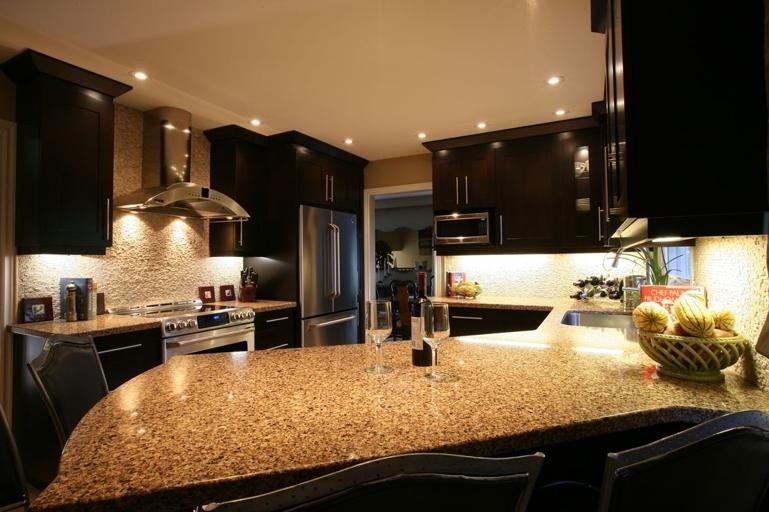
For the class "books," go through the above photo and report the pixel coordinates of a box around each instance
[59,276,97,320]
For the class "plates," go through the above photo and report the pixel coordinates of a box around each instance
[576,198,623,214]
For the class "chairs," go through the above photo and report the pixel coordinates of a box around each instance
[0,409,30,512]
[397,279,417,331]
[28,330,109,447]
[202,451,547,512]
[604,410,767,512]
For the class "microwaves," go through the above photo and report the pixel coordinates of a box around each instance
[435,212,493,247]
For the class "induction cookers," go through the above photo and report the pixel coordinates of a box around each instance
[115,299,249,318]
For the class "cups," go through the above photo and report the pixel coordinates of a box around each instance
[574,162,586,178]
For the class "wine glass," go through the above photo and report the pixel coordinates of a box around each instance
[366,301,392,376]
[421,303,450,381]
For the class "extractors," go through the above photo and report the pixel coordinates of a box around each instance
[114,106,250,222]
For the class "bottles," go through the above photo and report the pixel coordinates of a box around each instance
[65,281,78,322]
[411,271,433,366]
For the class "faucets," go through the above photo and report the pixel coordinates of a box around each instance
[610,238,694,268]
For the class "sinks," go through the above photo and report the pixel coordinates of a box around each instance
[560,307,639,331]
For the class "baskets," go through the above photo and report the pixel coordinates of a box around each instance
[638,330,745,384]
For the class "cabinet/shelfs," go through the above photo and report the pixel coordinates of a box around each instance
[204,129,364,210]
[424,301,489,344]
[12,327,162,434]
[255,299,297,353]
[591,0,686,235]
[423,129,505,213]
[2,48,133,258]
[506,118,580,250]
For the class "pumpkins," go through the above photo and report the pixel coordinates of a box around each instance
[711,305,736,332]
[631,300,670,334]
[706,328,736,340]
[663,321,683,338]
[671,294,715,338]
[684,289,706,305]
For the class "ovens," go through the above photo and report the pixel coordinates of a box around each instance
[162,307,255,364]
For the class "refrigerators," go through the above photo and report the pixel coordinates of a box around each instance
[299,205,364,347]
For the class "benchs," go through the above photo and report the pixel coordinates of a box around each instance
[377,299,394,340]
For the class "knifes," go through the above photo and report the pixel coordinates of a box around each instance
[240,266,258,288]
